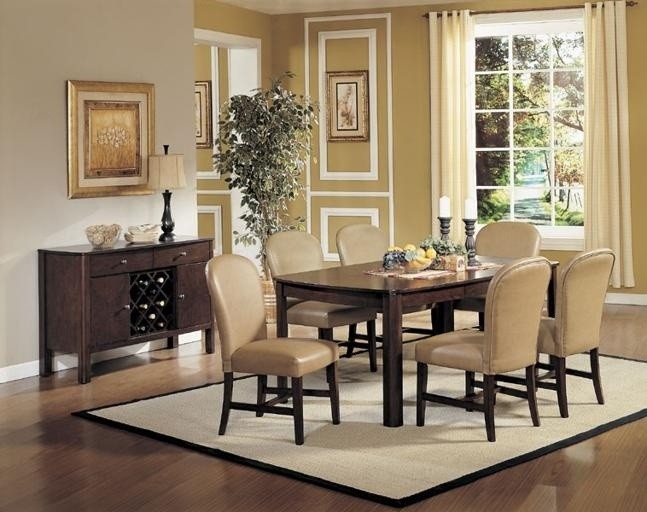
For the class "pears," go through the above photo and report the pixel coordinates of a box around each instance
[426,248,437,258]
[404,244,416,251]
[394,246,402,250]
[416,248,426,257]
[387,246,394,251]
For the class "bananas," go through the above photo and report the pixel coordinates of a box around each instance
[415,254,431,264]
[406,260,422,269]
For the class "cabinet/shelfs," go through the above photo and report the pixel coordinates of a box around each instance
[38,233,216,385]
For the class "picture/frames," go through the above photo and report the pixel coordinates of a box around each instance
[324,69,370,143]
[66,76,159,200]
[196,79,215,150]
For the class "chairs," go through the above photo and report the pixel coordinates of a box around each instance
[412,256,550,442]
[336,222,390,358]
[493,248,602,418]
[473,219,541,261]
[265,231,378,374]
[204,252,341,445]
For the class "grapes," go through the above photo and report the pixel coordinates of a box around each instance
[382,249,408,269]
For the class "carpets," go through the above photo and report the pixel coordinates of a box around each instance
[71,331,647,506]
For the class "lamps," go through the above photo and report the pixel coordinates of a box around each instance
[145,153,187,242]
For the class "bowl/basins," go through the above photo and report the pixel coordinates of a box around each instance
[395,259,434,274]
[86,225,123,250]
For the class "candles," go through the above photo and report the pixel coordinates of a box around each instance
[438,196,452,239]
[462,196,481,267]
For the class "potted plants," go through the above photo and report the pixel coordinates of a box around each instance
[209,69,320,325]
